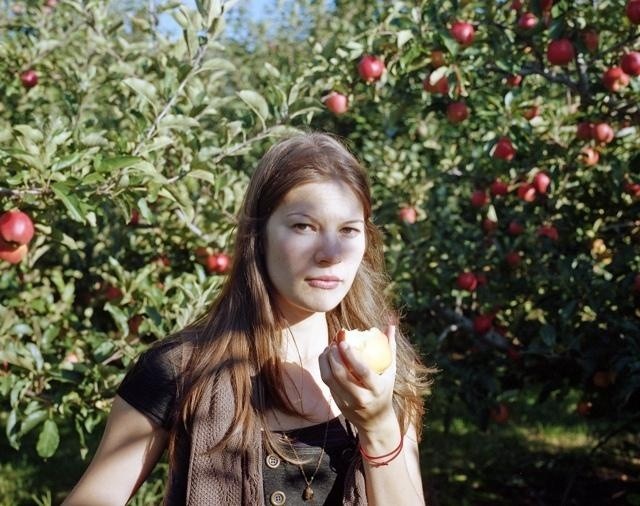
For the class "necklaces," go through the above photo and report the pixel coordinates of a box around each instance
[252,392,338,500]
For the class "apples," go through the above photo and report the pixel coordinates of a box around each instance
[1,0,640,425]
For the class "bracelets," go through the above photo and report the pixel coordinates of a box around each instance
[358,443,403,468]
[358,432,403,460]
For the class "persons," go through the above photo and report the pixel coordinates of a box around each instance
[59,130,445,506]
